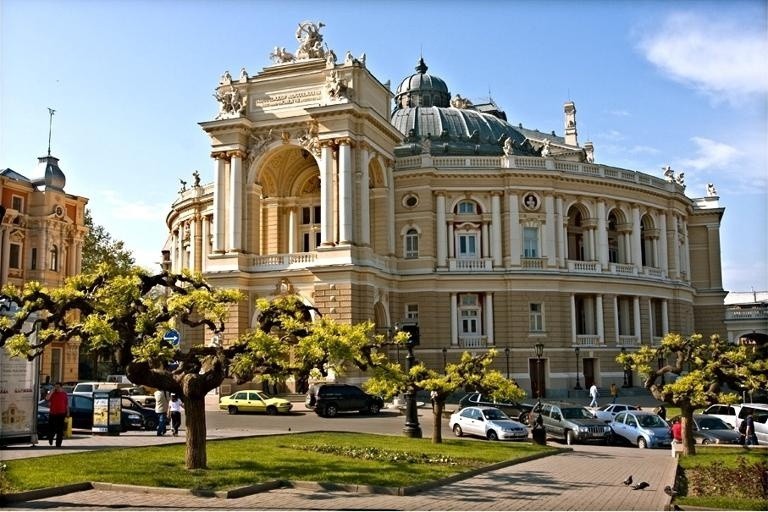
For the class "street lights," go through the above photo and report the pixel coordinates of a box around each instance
[573,347,584,390]
[533,337,545,402]
[504,346,511,380]
[622,346,632,388]
[441,346,449,377]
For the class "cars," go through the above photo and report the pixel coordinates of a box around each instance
[665,413,746,446]
[218,389,295,415]
[610,408,673,450]
[584,403,638,424]
[36,373,172,436]
[446,404,530,443]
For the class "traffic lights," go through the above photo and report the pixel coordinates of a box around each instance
[401,325,421,347]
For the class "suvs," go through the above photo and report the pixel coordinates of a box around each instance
[699,403,742,429]
[457,390,535,425]
[303,380,385,418]
[527,399,613,447]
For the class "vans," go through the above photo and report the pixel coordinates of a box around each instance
[735,402,768,446]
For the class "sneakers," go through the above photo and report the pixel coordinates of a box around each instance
[157,428,178,436]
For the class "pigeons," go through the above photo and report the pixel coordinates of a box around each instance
[664,486,678,496]
[620,475,633,486]
[630,482,650,490]
[674,505,684,511]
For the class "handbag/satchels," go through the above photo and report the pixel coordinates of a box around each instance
[63,417,73,439]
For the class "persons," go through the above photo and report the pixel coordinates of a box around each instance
[225,88,245,118]
[44,382,70,448]
[325,71,347,98]
[298,23,323,53]
[589,381,600,407]
[325,50,337,70]
[670,416,682,441]
[192,173,201,186]
[246,133,272,157]
[153,388,170,436]
[608,382,618,403]
[657,404,666,421]
[430,390,439,409]
[743,409,759,445]
[166,392,185,436]
